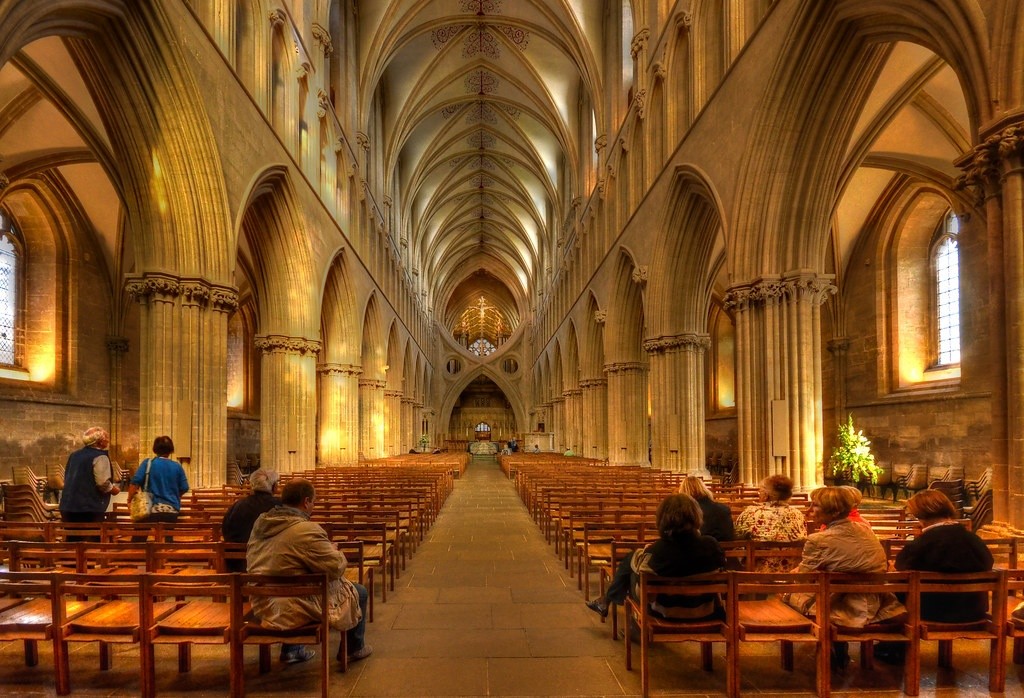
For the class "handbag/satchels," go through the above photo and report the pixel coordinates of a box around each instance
[130,457,152,522]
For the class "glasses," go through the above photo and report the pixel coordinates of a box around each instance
[758,490,765,495]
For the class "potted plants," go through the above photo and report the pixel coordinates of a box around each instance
[828,411,884,486]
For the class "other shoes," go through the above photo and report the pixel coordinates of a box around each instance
[874,641,905,666]
[830,642,850,668]
[337,644,373,662]
[620,627,641,644]
[280,649,316,663]
[584,600,609,617]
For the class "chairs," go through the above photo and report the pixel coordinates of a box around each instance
[496,451,1024,697]
[0,451,469,698]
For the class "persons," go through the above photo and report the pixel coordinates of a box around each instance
[246,478,373,663]
[433,448,440,453]
[534,445,540,453]
[127,436,189,543]
[734,474,807,583]
[585,493,727,636]
[785,486,888,669]
[820,485,871,531]
[507,440,518,452]
[409,447,419,454]
[59,427,121,542]
[875,490,994,666]
[222,465,281,575]
[564,447,574,456]
[679,476,739,566]
